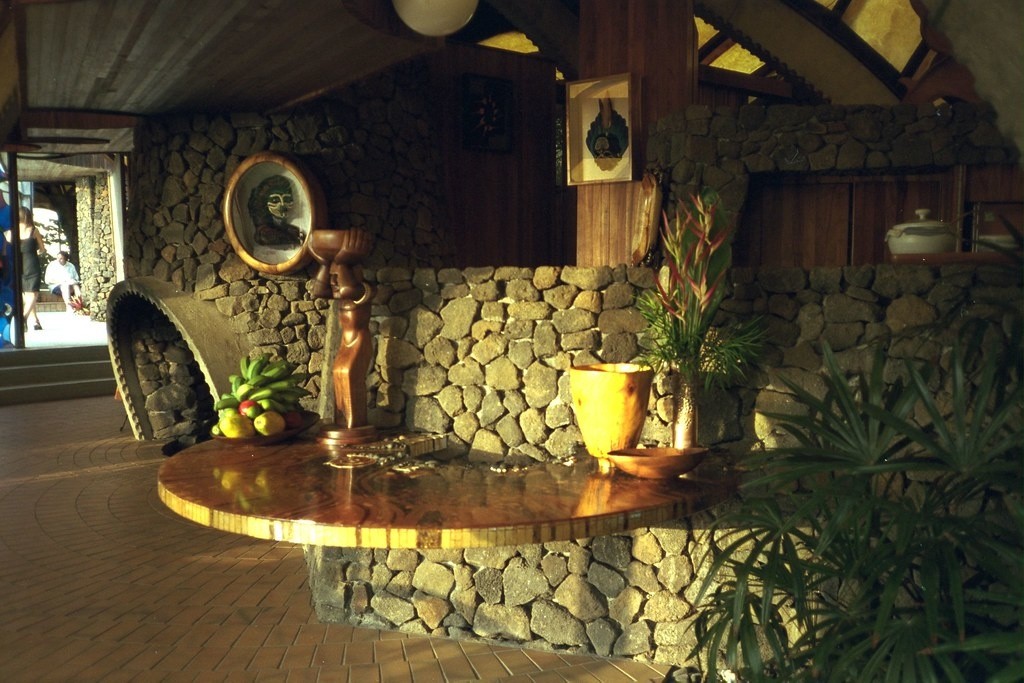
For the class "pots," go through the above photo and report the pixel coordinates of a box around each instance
[883,209,955,254]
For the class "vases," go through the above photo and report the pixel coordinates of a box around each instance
[672,380,699,448]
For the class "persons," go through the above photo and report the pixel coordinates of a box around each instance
[45,251,81,315]
[308,229,377,429]
[6,206,45,332]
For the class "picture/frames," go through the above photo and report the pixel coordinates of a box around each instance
[459,70,514,157]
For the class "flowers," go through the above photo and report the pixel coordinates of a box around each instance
[625,187,775,403]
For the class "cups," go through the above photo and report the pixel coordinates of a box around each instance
[569,363,652,472]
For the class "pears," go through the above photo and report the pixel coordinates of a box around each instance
[219,411,285,438]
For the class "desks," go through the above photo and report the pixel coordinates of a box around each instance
[156,427,739,551]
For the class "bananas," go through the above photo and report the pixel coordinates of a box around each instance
[212,352,313,415]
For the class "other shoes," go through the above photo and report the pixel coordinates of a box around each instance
[23,315,29,332]
[34,322,42,330]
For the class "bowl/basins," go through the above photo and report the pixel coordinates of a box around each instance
[608,447,709,478]
[313,229,372,264]
